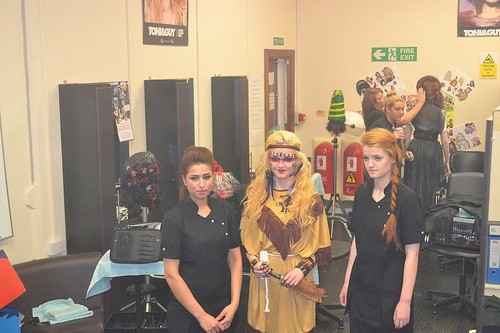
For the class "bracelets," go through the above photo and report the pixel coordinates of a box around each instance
[250,257,259,274]
[294,256,315,278]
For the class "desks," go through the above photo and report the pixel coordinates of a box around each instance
[316,240,351,330]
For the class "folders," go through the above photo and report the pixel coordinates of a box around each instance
[486,236,500,284]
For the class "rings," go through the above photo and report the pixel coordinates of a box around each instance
[262,269,265,273]
[265,274,267,277]
[264,272,266,275]
[261,265,263,270]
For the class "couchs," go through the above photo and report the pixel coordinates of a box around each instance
[7,252,102,333]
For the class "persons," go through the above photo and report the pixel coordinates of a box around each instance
[361,86,426,130]
[338,128,425,333]
[162,147,242,333]
[365,66,397,94]
[144,0,188,26]
[367,95,414,141]
[403,75,452,250]
[238,129,332,333]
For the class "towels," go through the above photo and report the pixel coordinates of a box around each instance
[312,172,325,196]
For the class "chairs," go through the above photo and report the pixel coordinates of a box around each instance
[421,150,484,321]
